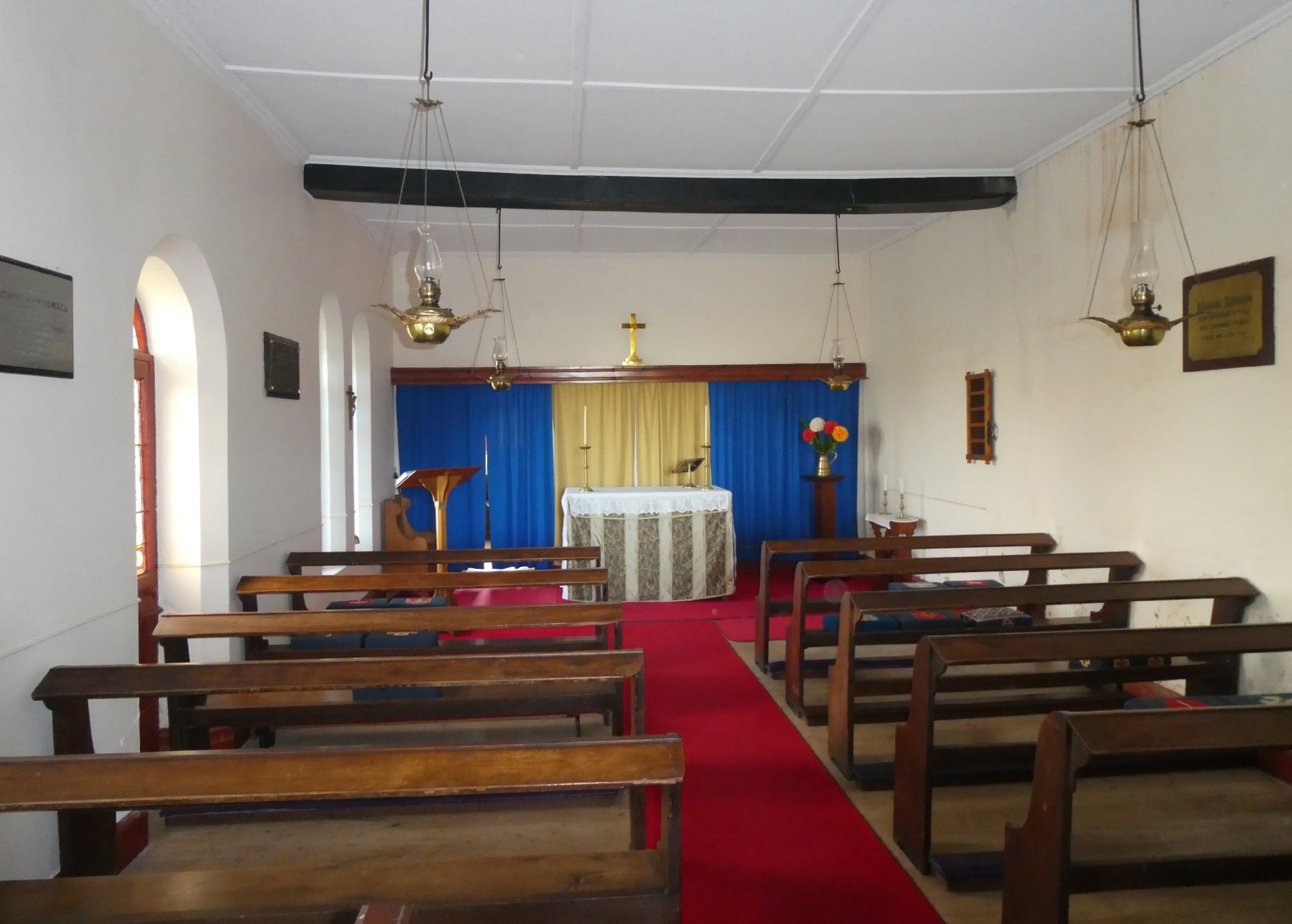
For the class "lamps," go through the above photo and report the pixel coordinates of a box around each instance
[370,1,503,345]
[472,206,534,390]
[812,212,869,390]
[1079,0,1209,347]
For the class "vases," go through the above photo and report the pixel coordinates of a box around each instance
[817,449,838,476]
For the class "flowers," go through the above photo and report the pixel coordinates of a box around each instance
[800,414,848,456]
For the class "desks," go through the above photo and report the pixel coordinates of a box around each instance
[561,485,737,603]
[866,513,919,582]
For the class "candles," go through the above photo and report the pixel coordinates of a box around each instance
[705,405,707,444]
[584,405,587,444]
[884,474,887,491]
[900,477,903,494]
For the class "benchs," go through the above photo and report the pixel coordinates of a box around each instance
[754,533,1291,924]
[1,545,685,923]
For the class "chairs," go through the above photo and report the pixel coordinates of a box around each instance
[384,493,437,598]
